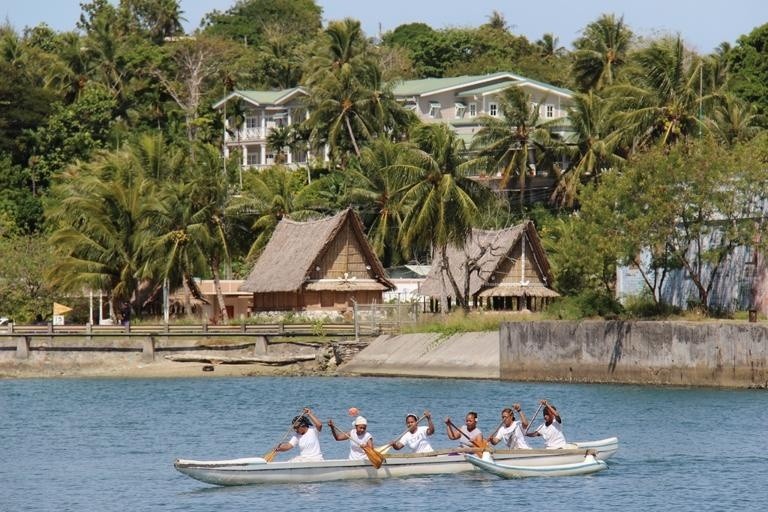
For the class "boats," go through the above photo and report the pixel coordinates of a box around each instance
[171,436,621,487]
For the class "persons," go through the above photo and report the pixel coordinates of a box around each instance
[522,398,568,448]
[120,301,130,326]
[327,414,375,459]
[442,411,483,447]
[274,406,325,462]
[487,402,531,450]
[388,408,436,453]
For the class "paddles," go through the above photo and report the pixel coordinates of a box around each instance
[447,421,494,458]
[264,409,307,463]
[332,423,385,468]
[374,416,425,455]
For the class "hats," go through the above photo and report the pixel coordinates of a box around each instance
[292,416,313,427]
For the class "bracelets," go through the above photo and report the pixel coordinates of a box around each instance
[517,408,521,412]
